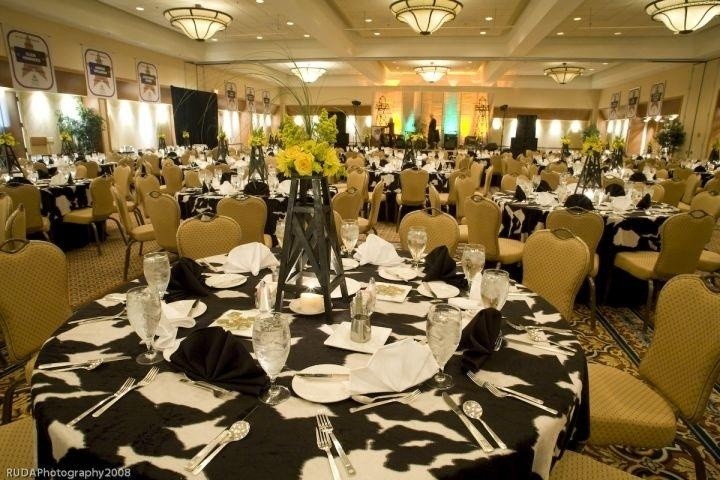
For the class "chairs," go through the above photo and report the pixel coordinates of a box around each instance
[0,412,38,479]
[455,150,720,328]
[273,148,456,261]
[548,449,655,480]
[0,236,79,416]
[587,273,719,478]
[1,126,273,267]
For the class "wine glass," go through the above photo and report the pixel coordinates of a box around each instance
[124,286,166,367]
[249,314,294,404]
[461,243,485,298]
[425,304,459,393]
[143,250,172,304]
[340,221,359,259]
[0,142,720,218]
[407,226,428,276]
[276,220,286,249]
[481,267,510,335]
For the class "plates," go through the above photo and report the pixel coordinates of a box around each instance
[163,257,486,364]
[291,362,353,403]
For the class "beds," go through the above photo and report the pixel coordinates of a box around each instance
[34,244,584,480]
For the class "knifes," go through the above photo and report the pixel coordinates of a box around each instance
[295,372,353,379]
[38,356,132,370]
[501,337,576,357]
[442,391,494,452]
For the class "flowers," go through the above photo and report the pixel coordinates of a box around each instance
[266,105,342,177]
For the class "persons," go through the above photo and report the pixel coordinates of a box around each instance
[386,119,397,147]
[379,127,388,147]
[427,114,436,149]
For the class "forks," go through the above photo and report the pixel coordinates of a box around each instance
[349,388,422,414]
[314,407,358,478]
[66,376,135,428]
[92,366,160,419]
[315,427,342,479]
[467,369,544,405]
[506,319,573,334]
[178,377,227,402]
[484,382,559,415]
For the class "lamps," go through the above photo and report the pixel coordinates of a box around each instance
[414,67,450,85]
[390,0,464,36]
[291,67,326,83]
[643,0,718,36]
[163,4,236,43]
[543,63,586,87]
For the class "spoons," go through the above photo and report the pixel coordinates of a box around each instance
[51,358,104,371]
[350,391,410,405]
[462,399,508,450]
[183,420,250,472]
[527,330,578,354]
